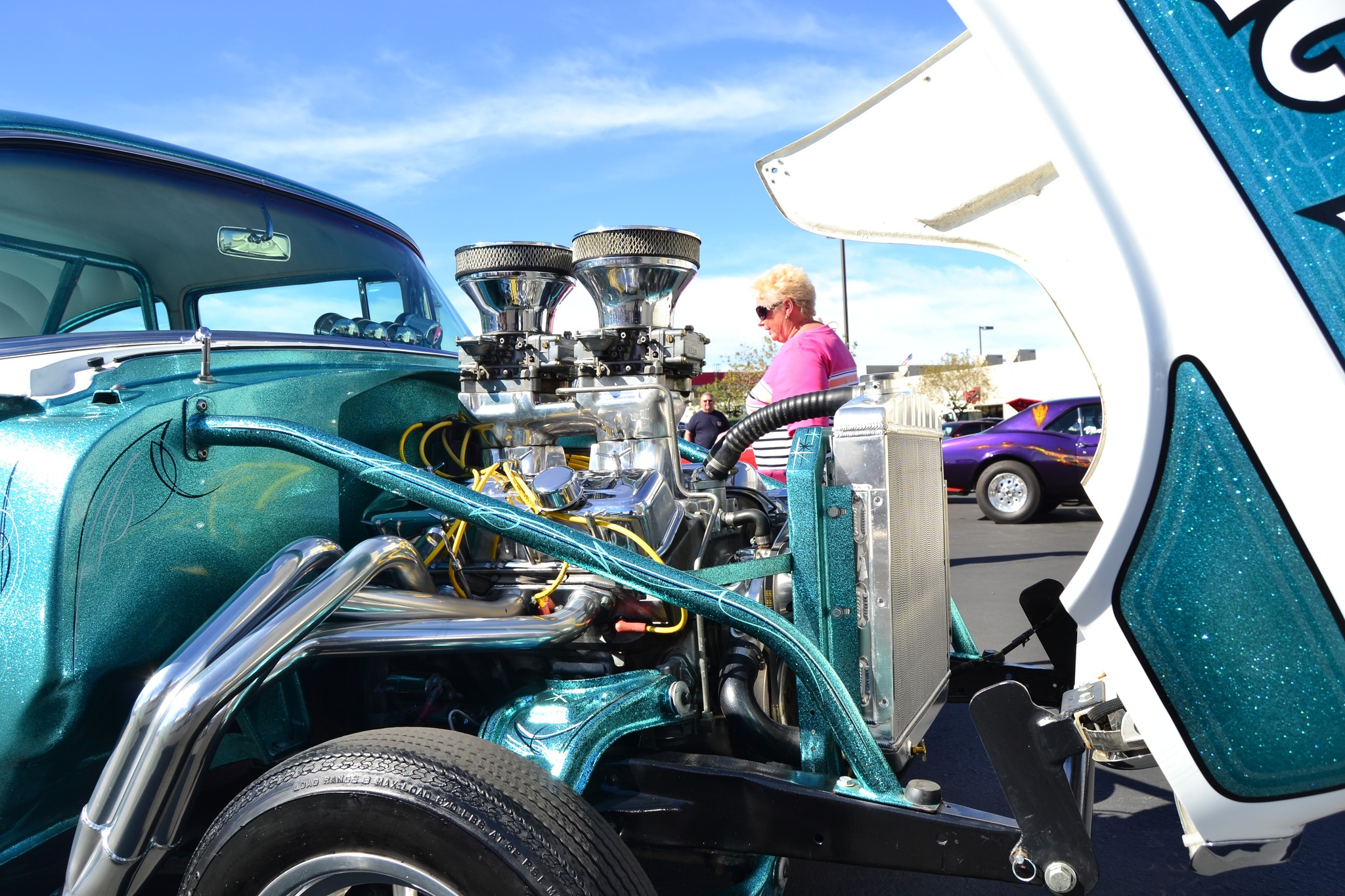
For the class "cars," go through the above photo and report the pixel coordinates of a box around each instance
[941,396,1103,525]
[0,0,1345,896]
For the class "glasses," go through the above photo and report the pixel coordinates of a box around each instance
[756,299,784,320]
[701,399,714,403]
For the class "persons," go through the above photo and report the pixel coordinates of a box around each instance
[744,263,858,470]
[684,391,730,450]
